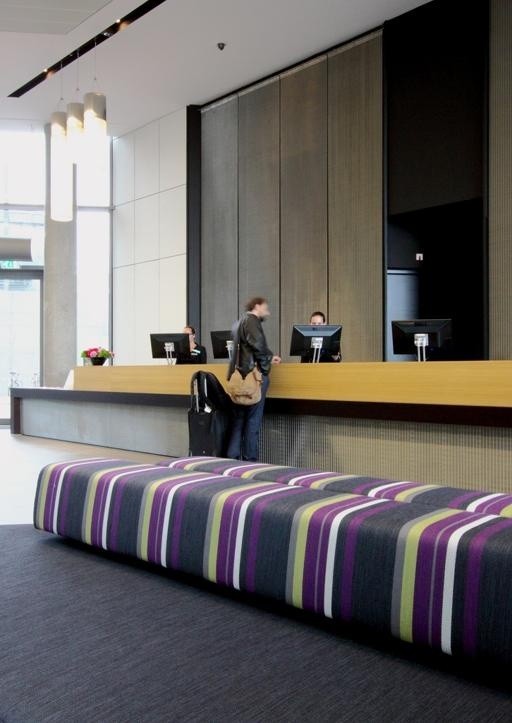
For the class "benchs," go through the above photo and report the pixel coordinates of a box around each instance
[32,454,512,675]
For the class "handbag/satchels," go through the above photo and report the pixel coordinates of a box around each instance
[225,361,264,406]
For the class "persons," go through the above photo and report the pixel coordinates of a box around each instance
[223,296,282,462]
[174,326,208,364]
[299,309,344,362]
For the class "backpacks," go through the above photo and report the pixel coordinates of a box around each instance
[190,371,233,414]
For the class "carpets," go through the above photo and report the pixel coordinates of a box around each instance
[0,523,512,722]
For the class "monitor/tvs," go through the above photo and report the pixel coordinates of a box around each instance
[211,330,233,359]
[150,334,191,365]
[290,325,342,363]
[391,318,452,361]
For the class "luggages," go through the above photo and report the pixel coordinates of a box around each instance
[185,373,229,459]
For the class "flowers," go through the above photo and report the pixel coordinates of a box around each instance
[81,347,114,359]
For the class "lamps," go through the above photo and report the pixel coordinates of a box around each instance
[50,37,106,141]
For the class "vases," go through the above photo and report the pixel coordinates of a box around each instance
[90,357,106,365]
